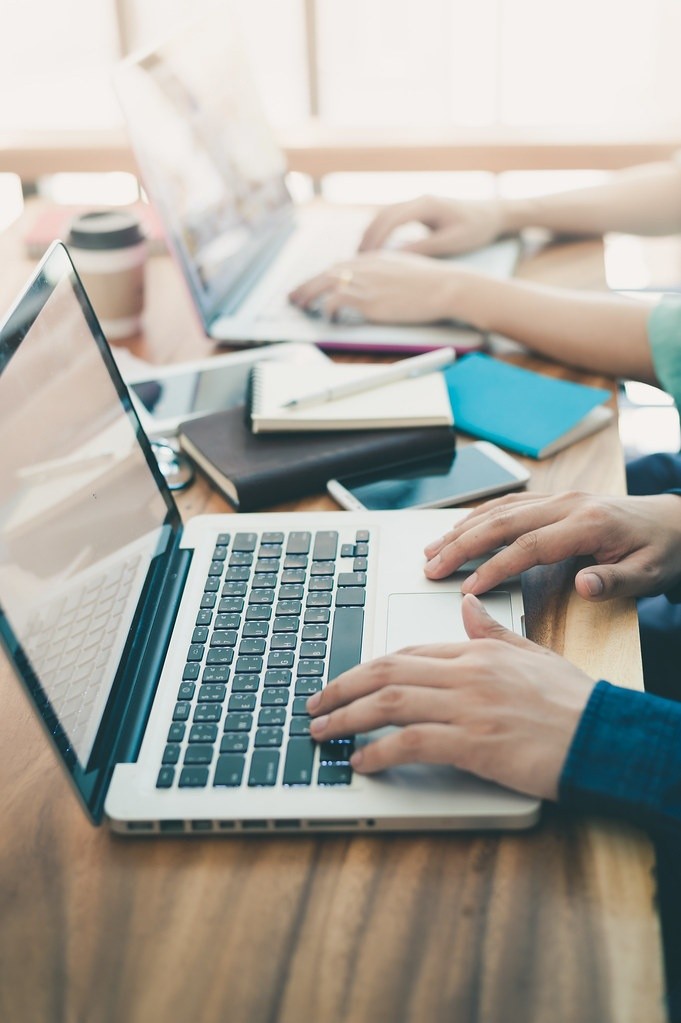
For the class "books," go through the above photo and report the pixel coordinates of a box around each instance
[444,354,615,461]
[175,406,456,513]
[243,364,454,434]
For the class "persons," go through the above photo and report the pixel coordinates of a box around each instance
[287,161,681,495]
[305,487,681,842]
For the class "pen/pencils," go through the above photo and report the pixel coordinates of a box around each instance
[280,345,458,414]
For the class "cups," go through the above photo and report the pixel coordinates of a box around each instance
[68,211,148,340]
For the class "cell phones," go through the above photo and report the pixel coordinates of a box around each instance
[324,439,532,512]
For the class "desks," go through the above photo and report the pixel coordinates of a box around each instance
[0,205,670,1023]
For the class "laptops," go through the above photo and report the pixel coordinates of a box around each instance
[122,54,522,358]
[0,240,542,833]
